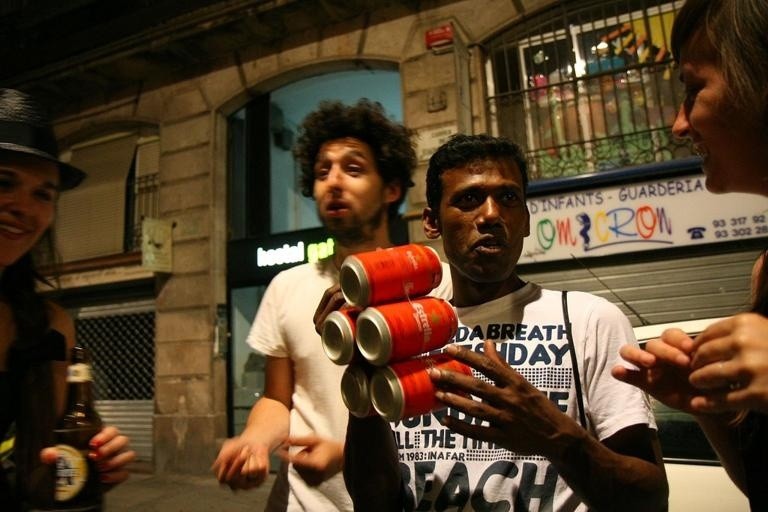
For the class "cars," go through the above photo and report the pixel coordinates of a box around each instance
[631,312,753,512]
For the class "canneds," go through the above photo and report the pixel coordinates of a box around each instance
[321,305,355,364]
[370,352,473,420]
[341,365,380,416]
[355,298,458,366]
[339,244,443,309]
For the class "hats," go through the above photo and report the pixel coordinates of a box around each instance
[0,86,87,190]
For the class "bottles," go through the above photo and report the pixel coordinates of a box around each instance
[50,344,105,511]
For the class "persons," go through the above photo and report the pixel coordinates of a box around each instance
[211,93,453,512]
[311,129,671,512]
[0,85,141,511]
[605,0,767,512]
